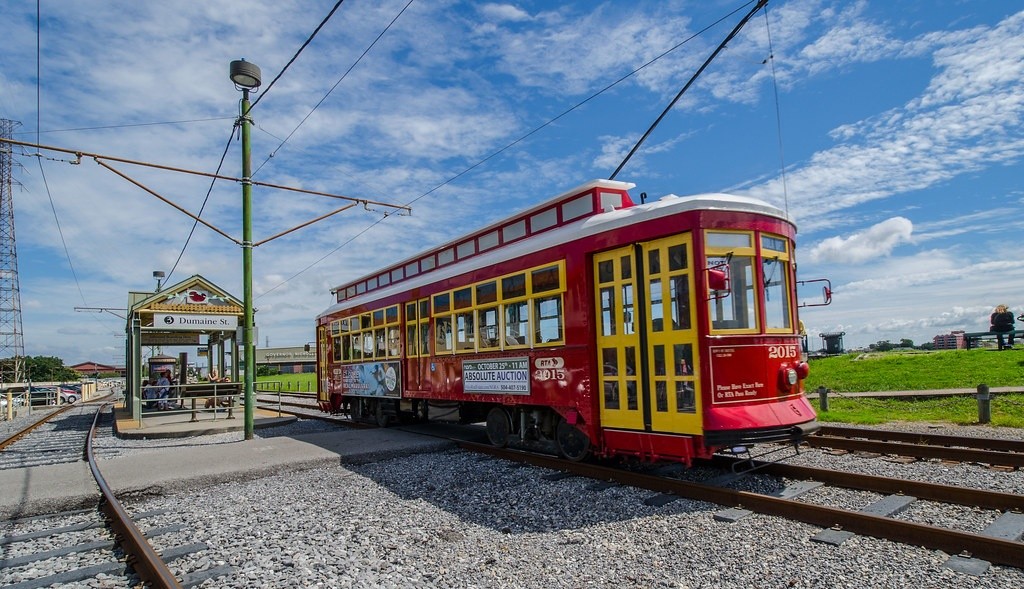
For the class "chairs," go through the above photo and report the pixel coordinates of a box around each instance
[354,348,393,359]
[651,318,682,379]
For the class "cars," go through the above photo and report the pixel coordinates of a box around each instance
[0,384,82,406]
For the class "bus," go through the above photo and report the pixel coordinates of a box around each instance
[304,178,834,476]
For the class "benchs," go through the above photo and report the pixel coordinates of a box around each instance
[963,330,1024,350]
[178,381,242,411]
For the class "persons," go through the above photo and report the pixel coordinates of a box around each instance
[142,369,177,411]
[990,304,1015,350]
[208,369,219,384]
[205,377,231,409]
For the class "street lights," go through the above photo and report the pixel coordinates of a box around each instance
[229,57,261,442]
